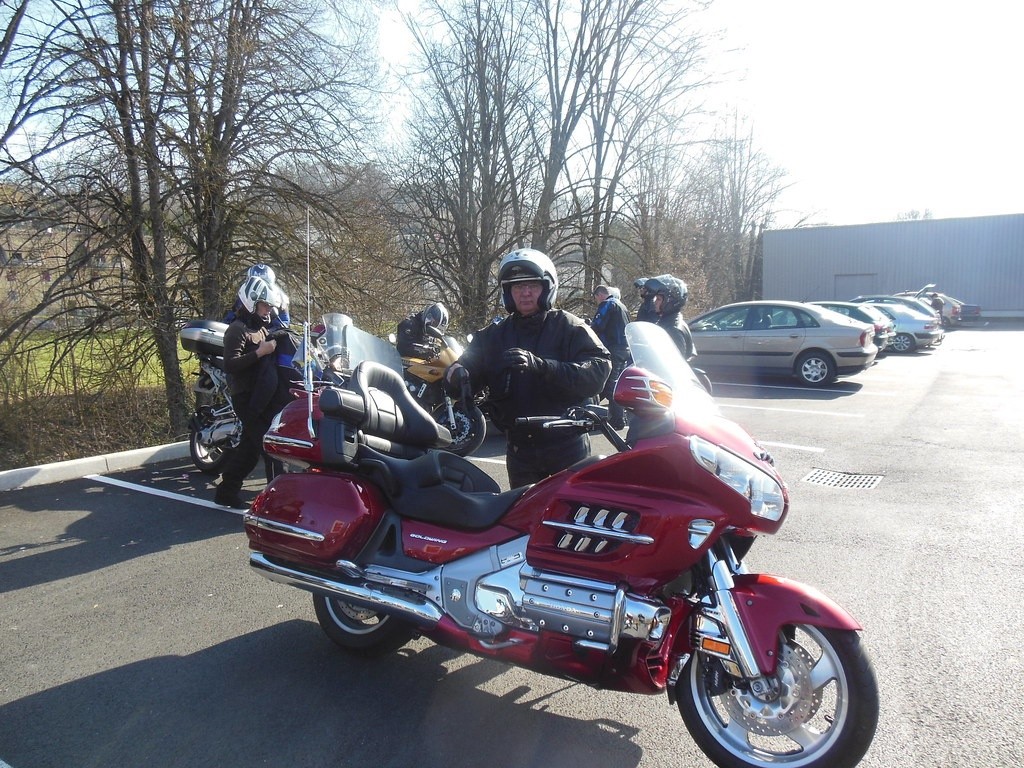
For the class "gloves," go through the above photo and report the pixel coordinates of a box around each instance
[503,347,544,381]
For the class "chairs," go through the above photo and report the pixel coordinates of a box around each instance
[762,314,774,328]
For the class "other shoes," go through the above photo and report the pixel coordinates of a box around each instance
[223,494,251,510]
[606,418,626,430]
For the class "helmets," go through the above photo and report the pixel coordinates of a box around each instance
[236,276,281,314]
[640,274,688,315]
[633,277,650,299]
[423,303,448,333]
[498,248,558,314]
[247,265,275,284]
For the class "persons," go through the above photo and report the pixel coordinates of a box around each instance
[645,274,695,366]
[393,302,449,360]
[930,293,943,318]
[633,278,659,324]
[215,276,295,510]
[589,283,633,429]
[220,265,292,329]
[443,247,613,490]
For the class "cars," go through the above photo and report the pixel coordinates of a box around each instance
[728,301,897,354]
[851,283,980,353]
[684,300,878,388]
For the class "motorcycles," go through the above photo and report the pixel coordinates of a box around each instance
[244,206,879,768]
[386,334,489,457]
[180,313,353,478]
